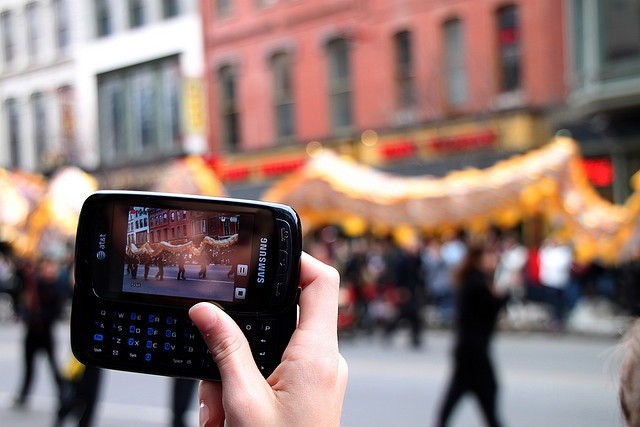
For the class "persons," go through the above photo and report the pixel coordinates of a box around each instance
[126,239,238,283]
[188,249,348,427]
[433,234,506,427]
[350,215,574,351]
[5,261,104,426]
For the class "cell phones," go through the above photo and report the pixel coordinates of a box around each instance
[66,190,302,387]
